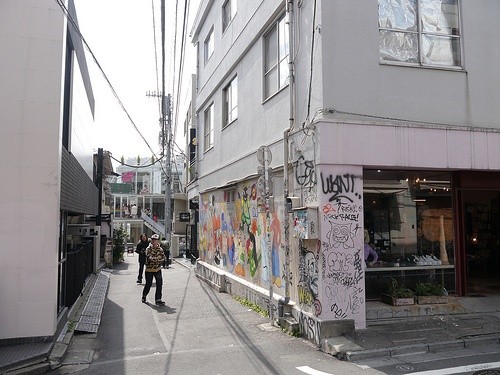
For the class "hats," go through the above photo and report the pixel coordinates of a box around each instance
[151,234,160,240]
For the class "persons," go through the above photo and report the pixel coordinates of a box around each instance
[110,201,157,224]
[160,238,171,269]
[141,233,165,306]
[135,233,152,284]
[139,184,150,195]
[364,228,378,268]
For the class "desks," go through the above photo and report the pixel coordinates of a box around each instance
[365,259,455,300]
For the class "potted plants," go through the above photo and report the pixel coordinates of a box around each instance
[411,281,449,303]
[383,278,415,306]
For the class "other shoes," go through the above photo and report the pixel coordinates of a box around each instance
[142,297,146,302]
[137,279,142,283]
[155,301,165,305]
